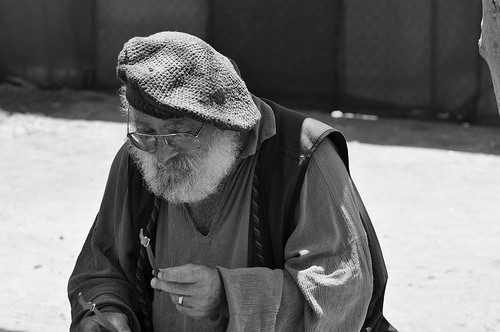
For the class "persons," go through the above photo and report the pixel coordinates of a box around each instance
[67,31,398,332]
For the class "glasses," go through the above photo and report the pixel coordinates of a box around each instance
[127,117,205,153]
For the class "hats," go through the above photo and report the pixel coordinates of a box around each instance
[116,31,261,132]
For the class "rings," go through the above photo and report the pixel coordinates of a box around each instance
[178,296,184,306]
